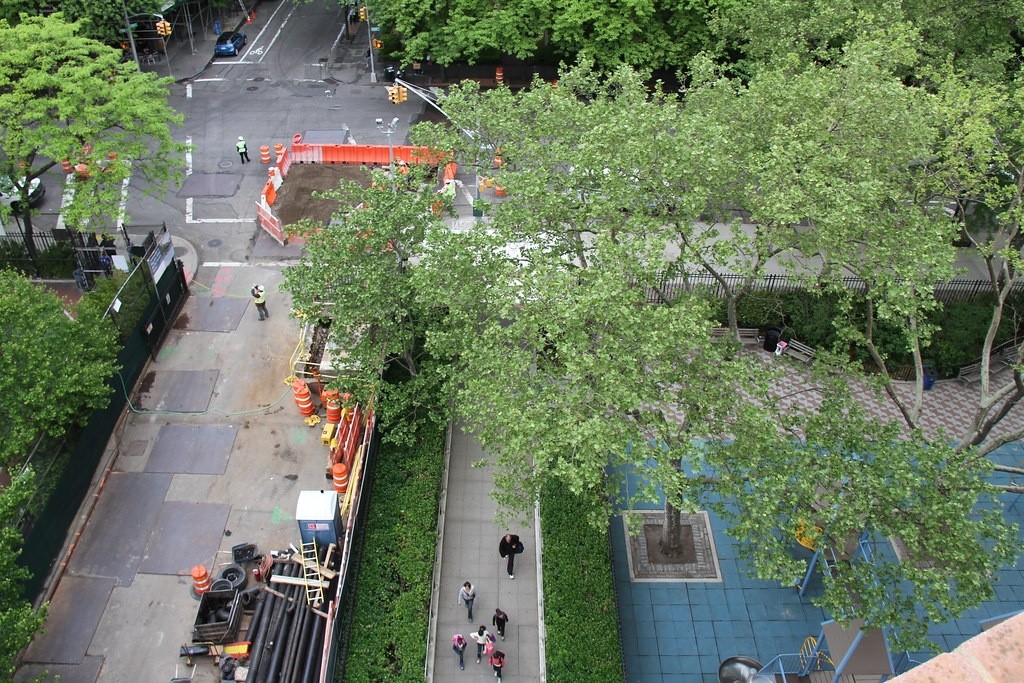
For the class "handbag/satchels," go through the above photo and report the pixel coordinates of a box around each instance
[514,542,526,554]
[472,594,475,599]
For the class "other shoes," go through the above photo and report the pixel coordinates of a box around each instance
[501,636,505,641]
[258,318,265,321]
[460,667,464,671]
[476,659,481,664]
[497,678,501,683]
[468,618,473,623]
[494,671,497,677]
[497,631,501,635]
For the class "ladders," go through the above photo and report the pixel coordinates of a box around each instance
[298,537,324,605]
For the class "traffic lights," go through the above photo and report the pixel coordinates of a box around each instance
[391,87,399,105]
[159,24,165,36]
[156,23,160,35]
[165,22,172,35]
[398,86,408,103]
[376,42,384,49]
[359,7,366,21]
[121,41,128,51]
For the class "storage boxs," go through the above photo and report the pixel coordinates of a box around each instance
[193,589,242,644]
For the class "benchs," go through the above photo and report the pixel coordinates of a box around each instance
[958,343,1020,388]
[784,339,844,375]
[712,328,760,344]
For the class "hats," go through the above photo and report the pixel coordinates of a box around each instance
[258,286,265,292]
[238,136,244,141]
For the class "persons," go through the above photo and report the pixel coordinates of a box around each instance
[443,179,454,198]
[499,534,519,579]
[236,136,251,164]
[399,161,408,176]
[453,637,467,670]
[470,626,489,663]
[251,284,269,320]
[459,582,479,623]
[489,652,505,683]
[87,232,116,269]
[365,46,370,68]
[493,609,509,640]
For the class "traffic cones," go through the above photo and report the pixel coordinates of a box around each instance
[251,8,257,20]
[247,15,253,25]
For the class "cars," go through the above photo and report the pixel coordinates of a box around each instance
[0,173,45,215]
[214,30,247,58]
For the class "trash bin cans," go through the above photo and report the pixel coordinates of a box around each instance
[72,269,84,291]
[763,327,782,352]
[923,365,938,391]
[775,341,788,358]
